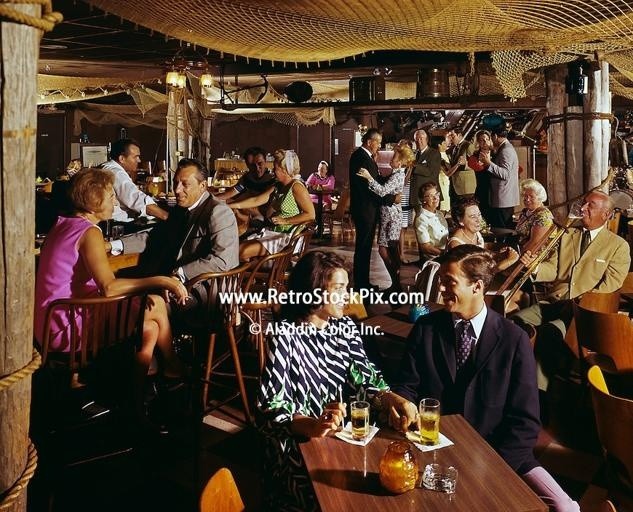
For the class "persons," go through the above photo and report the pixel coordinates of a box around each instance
[513,177,561,285]
[504,190,631,424]
[137,158,241,335]
[349,124,531,318]
[394,244,543,476]
[33,169,176,385]
[253,249,420,512]
[31,143,336,273]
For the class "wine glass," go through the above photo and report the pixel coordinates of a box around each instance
[35,234,47,249]
[151,186,159,201]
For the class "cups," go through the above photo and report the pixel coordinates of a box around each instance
[167,196,177,206]
[349,401,371,442]
[419,398,441,446]
[226,153,230,160]
[112,225,124,238]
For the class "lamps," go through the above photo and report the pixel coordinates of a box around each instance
[165,41,213,89]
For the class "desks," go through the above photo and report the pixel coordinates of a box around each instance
[103,209,250,274]
[214,158,274,172]
[298,413,550,512]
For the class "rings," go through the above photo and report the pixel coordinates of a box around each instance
[323,412,335,422]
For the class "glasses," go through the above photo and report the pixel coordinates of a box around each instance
[424,192,440,200]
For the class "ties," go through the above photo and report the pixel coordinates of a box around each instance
[580,231,591,257]
[456,320,472,378]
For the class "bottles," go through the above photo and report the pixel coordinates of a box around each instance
[121,127,126,139]
[408,301,430,323]
[83,134,88,143]
[268,155,272,162]
[380,443,419,494]
[110,226,124,258]
[104,220,112,258]
[212,173,242,187]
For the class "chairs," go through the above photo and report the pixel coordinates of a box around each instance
[322,189,355,242]
[41,290,148,371]
[574,292,633,373]
[199,468,245,512]
[586,363,633,491]
